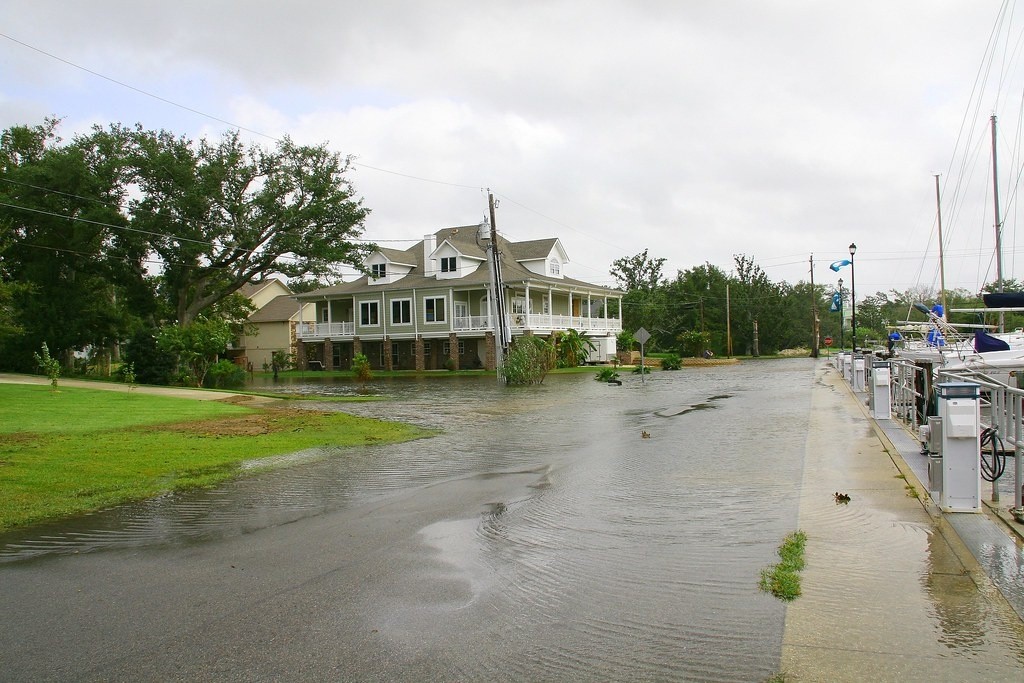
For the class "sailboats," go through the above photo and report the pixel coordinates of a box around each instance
[885,0,1024,392]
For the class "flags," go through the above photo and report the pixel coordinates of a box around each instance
[830,291,842,312]
[830,260,849,272]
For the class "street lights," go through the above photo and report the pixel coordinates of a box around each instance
[838,277,844,351]
[848,242,857,353]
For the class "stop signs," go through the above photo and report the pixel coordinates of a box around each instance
[824,337,832,346]
